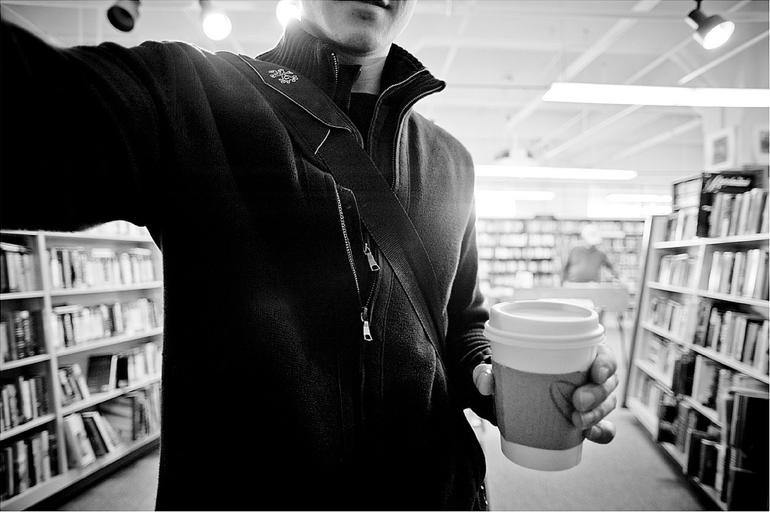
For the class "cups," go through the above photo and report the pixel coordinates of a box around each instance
[482,298,605,472]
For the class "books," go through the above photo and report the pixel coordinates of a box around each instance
[2,242,60,501]
[683,176,769,510]
[619,163,699,450]
[53,246,164,470]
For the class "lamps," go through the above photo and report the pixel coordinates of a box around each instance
[685,1,737,50]
[106,0,141,32]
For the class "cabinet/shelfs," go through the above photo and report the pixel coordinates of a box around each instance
[0,219,164,510]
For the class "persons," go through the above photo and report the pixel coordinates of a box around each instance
[564,225,617,285]
[2,2,621,509]
